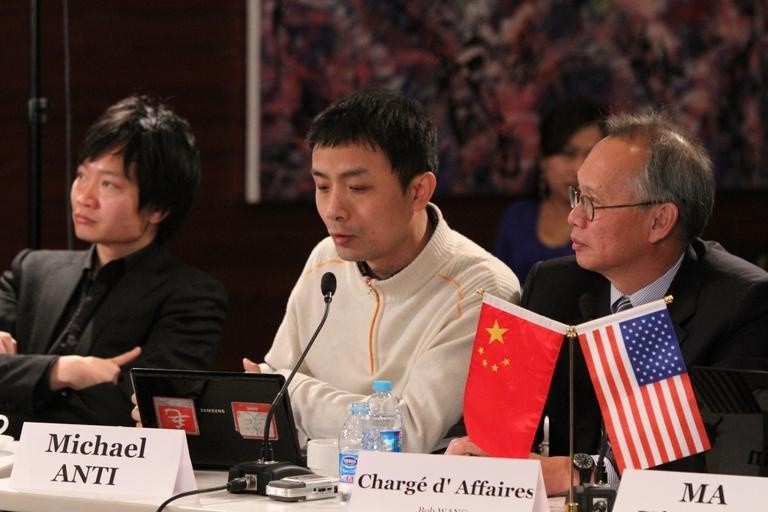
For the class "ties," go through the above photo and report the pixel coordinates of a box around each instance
[600,296,634,463]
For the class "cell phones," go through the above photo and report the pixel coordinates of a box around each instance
[266,474,338,502]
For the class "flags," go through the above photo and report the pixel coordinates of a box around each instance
[570,300,710,476]
[460,292,562,459]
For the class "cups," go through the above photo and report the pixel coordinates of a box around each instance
[305,437,341,476]
[0,415,11,434]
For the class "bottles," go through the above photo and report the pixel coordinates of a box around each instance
[366,376,403,449]
[337,399,380,503]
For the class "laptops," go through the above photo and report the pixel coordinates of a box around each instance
[129,366,307,471]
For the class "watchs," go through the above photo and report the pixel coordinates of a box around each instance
[571,452,595,486]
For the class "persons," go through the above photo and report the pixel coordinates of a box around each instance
[127,85,521,453]
[431,106,766,481]
[0,93,231,446]
[491,94,618,295]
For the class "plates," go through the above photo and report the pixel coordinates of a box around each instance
[0,435,15,448]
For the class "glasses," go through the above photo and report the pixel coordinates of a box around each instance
[568,185,671,221]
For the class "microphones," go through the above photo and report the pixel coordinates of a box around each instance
[565,292,617,512]
[228,272,337,496]
